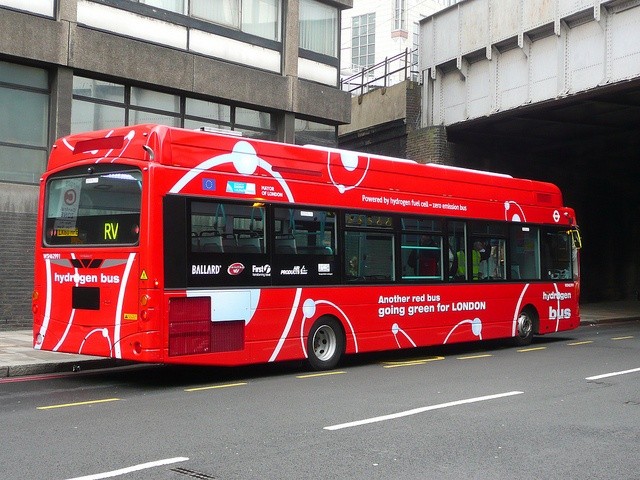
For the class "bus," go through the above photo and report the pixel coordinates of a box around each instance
[32,123,584,371]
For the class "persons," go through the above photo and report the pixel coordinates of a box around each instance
[408,235,441,282]
[473,242,495,279]
[449,243,492,281]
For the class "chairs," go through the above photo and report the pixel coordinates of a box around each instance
[199,230,225,253]
[237,231,261,253]
[221,232,237,252]
[192,231,198,252]
[275,233,297,254]
